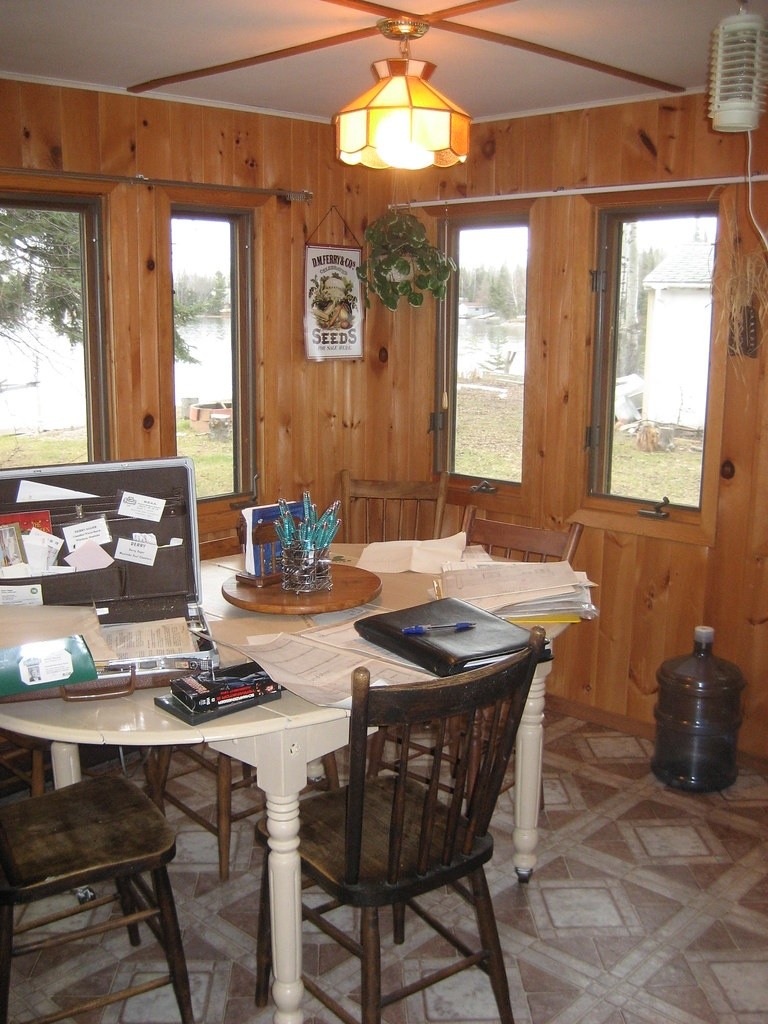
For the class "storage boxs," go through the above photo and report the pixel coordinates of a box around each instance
[0,635,99,698]
[0,456,221,703]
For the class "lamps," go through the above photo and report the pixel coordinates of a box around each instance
[331,19,474,171]
[707,4,768,132]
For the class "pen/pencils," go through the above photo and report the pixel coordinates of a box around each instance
[278,498,299,541]
[273,519,284,545]
[402,621,478,635]
[282,511,292,548]
[298,490,343,583]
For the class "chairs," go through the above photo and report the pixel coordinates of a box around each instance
[367,503,584,811]
[255,624,546,1024]
[0,727,341,884]
[0,776,193,1024]
[340,469,450,544]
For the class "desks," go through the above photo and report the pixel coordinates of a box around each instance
[0,543,573,1024]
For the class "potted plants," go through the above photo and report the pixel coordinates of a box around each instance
[357,211,457,313]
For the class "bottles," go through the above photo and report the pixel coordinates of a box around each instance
[649,625,748,792]
